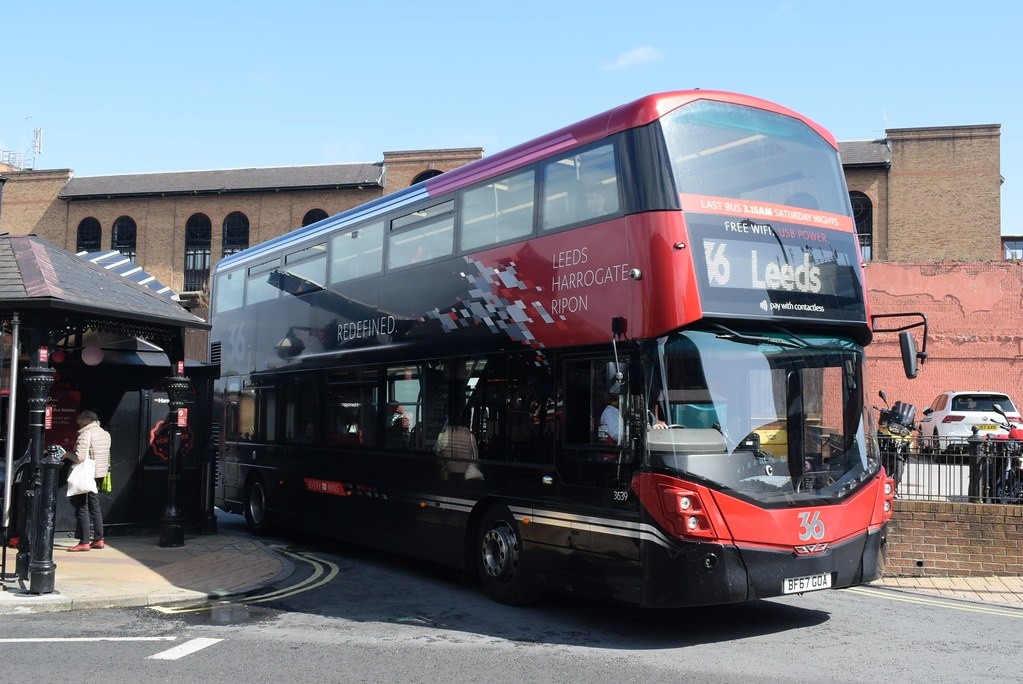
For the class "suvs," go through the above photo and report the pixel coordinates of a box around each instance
[916,388,1023,462]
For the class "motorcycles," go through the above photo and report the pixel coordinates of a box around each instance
[873,390,935,500]
[978,404,1023,506]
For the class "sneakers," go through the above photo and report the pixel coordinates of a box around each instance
[68,543,90,551]
[91,541,104,549]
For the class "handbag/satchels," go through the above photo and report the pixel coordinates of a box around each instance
[100,472,112,492]
[67,459,98,496]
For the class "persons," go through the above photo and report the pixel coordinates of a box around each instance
[295,421,315,443]
[385,418,410,438]
[598,395,670,458]
[62,411,111,551]
[8,428,49,549]
[435,408,480,499]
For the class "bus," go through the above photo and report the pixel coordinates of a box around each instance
[205,88,931,607]
